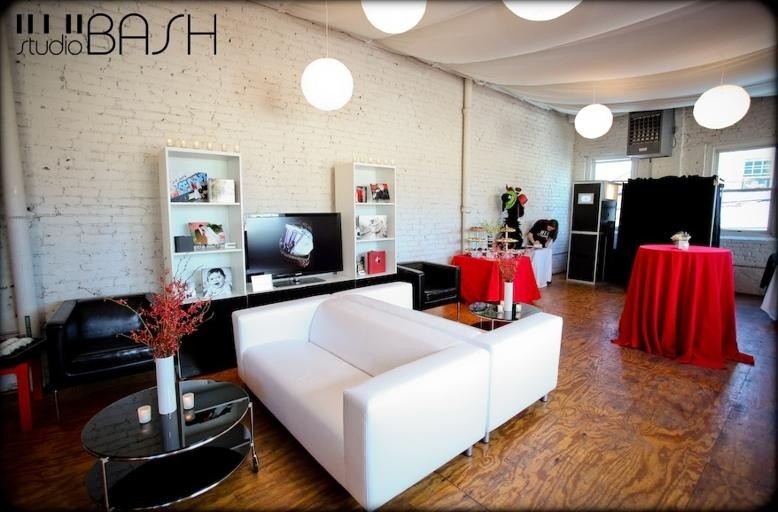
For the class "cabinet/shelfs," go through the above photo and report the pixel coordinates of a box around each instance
[158,148,248,307]
[176,275,398,379]
[334,162,397,281]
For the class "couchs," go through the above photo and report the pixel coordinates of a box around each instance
[231,294,493,512]
[332,281,564,442]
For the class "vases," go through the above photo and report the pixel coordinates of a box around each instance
[503,282,513,320]
[154,355,177,415]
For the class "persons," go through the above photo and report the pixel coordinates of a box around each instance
[528,219,558,249]
[203,267,232,299]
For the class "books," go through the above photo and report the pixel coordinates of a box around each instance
[364,250,386,275]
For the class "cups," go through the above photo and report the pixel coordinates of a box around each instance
[497,300,521,312]
[183,394,194,410]
[137,405,151,424]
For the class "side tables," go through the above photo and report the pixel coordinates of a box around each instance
[0,337,48,433]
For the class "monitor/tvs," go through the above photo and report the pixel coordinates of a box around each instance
[243,212,344,288]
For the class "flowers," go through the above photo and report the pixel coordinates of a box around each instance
[76,252,220,358]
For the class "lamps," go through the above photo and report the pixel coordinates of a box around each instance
[574,84,614,139]
[693,67,751,129]
[359,0,427,35]
[300,0,354,111]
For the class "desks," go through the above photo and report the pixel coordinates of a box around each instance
[468,302,543,331]
[610,244,755,370]
[465,247,552,289]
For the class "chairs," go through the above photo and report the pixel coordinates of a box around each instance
[396,261,460,322]
[46,292,168,423]
